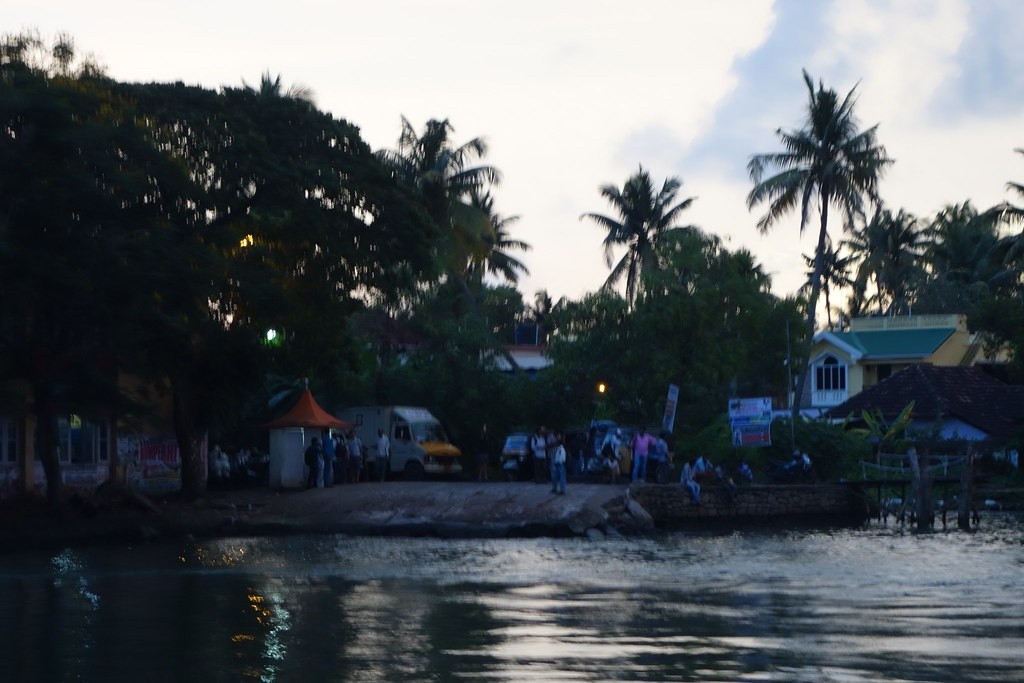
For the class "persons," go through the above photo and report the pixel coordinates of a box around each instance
[211,427,818,504]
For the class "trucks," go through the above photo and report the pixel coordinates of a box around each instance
[330,405,462,481]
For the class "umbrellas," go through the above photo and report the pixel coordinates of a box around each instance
[263,389,351,489]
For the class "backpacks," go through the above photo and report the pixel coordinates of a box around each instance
[305,448,316,465]
[336,443,344,457]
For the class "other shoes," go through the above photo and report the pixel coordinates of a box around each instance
[631,480,645,484]
[549,489,566,496]
[691,499,700,504]
